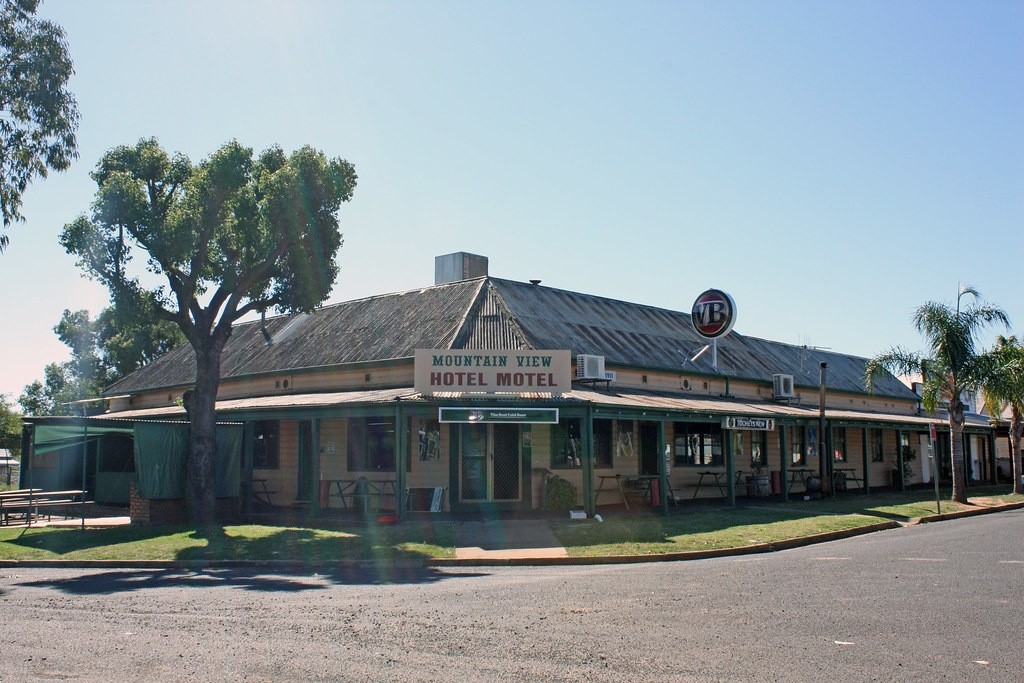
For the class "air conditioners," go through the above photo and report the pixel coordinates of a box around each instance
[772,373,795,400]
[577,353,606,377]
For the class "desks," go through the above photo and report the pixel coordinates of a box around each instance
[833,468,862,493]
[329,478,395,512]
[787,469,817,495]
[0,489,90,525]
[0,488,43,521]
[692,469,750,502]
[596,472,679,507]
[253,477,271,506]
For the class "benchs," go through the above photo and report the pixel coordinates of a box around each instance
[256,490,278,494]
[331,492,416,497]
[595,484,679,493]
[846,476,864,493]
[0,497,95,507]
[688,482,753,488]
[787,479,808,495]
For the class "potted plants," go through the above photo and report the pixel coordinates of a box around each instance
[746,454,772,498]
[893,445,918,487]
